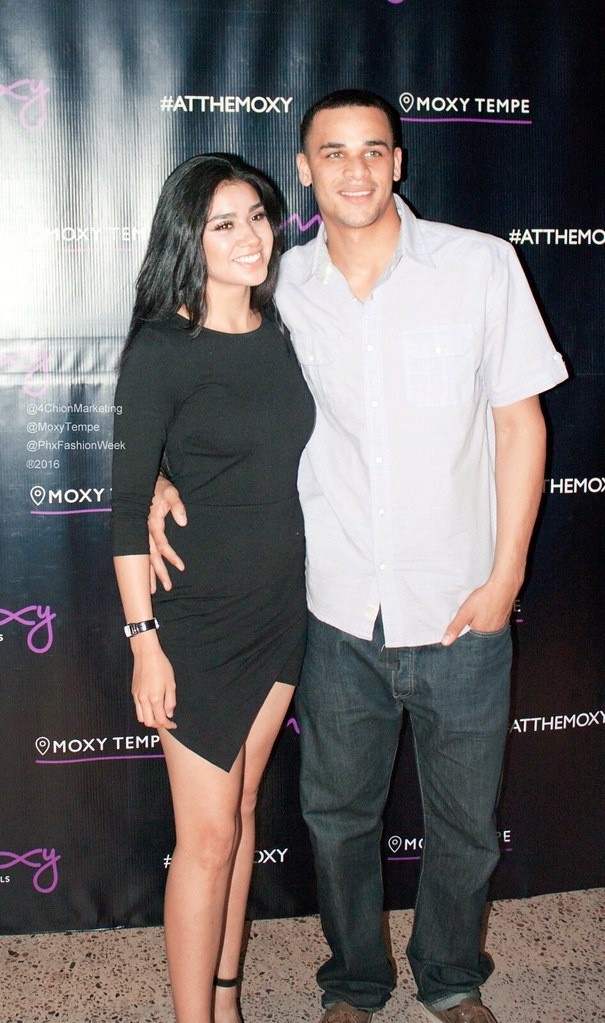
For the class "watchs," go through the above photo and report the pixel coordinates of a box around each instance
[124,617,159,638]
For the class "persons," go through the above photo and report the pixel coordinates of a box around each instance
[145,85,567,1023]
[106,155,318,1023]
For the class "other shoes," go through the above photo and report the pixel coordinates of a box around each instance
[319,1001,372,1023]
[417,993,498,1023]
